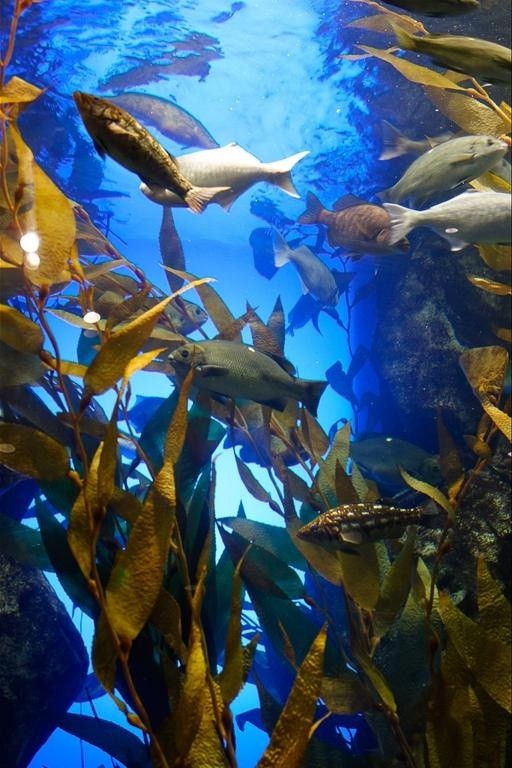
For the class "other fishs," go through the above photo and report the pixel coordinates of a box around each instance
[70,87,512,337]
[295,502,433,551]
